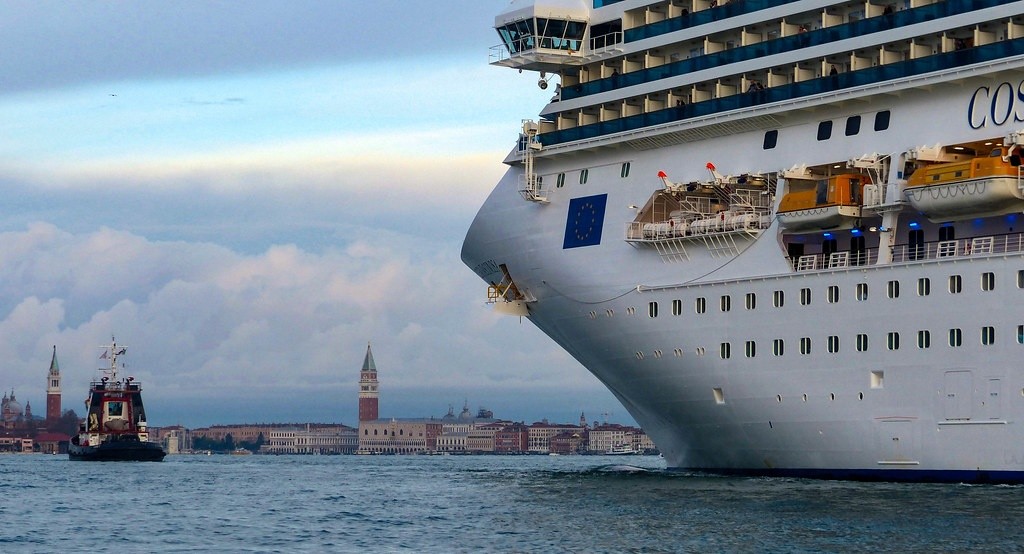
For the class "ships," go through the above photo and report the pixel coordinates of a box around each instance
[457,2,1024,484]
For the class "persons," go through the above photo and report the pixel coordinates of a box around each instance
[725,0,733,18]
[676,99,685,120]
[745,80,765,105]
[829,65,838,90]
[513,32,528,51]
[798,25,809,48]
[611,69,620,89]
[680,8,689,28]
[708,1,717,21]
[955,39,968,50]
[883,3,894,29]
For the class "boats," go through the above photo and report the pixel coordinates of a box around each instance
[68,334,168,462]
[642,221,691,237]
[774,175,877,231]
[691,212,723,234]
[722,214,770,228]
[902,158,1024,215]
[604,443,639,455]
[549,453,561,456]
